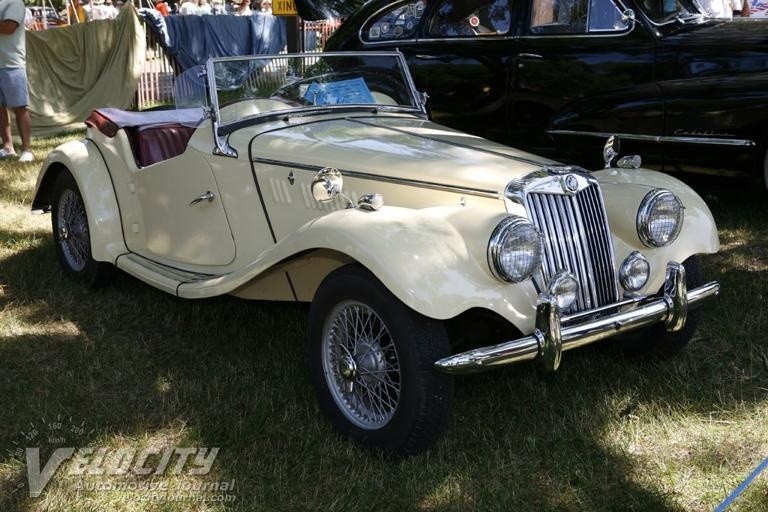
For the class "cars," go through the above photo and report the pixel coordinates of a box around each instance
[31,0,767,460]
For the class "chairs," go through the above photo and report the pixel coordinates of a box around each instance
[141,121,198,165]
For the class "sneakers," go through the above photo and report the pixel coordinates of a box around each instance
[0,145,35,162]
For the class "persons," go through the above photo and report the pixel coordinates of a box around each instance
[692,0,750,18]
[0,0,34,162]
[78,0,272,23]
